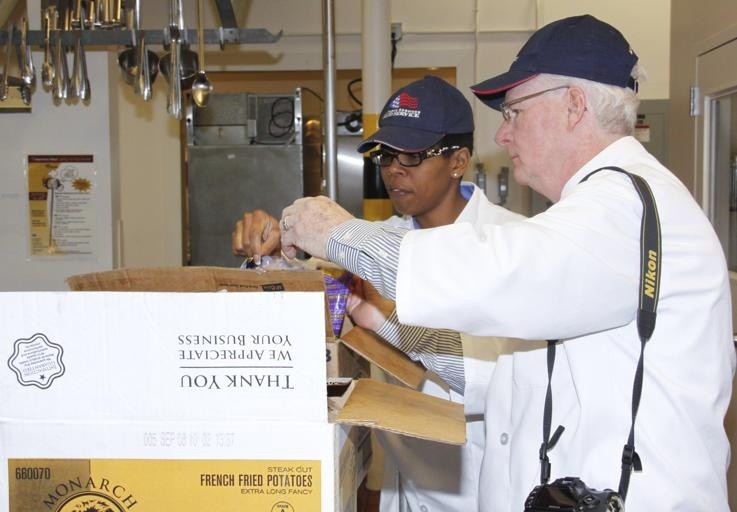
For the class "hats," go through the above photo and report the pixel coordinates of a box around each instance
[355,73,476,154]
[467,12,640,115]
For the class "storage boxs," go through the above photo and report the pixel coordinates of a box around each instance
[67,272,427,498]
[1,290,467,512]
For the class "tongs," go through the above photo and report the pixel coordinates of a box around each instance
[0,1,36,108]
[162,25,186,123]
[130,27,155,103]
[40,1,92,101]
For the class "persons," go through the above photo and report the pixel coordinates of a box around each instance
[275,13,734,511]
[232,76,545,511]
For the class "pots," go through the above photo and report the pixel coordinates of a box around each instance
[159,29,201,90]
[118,0,160,88]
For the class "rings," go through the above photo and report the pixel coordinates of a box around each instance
[282,216,288,231]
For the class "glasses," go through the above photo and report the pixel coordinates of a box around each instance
[368,144,462,168]
[498,84,588,125]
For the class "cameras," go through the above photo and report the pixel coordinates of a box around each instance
[523,476,626,512]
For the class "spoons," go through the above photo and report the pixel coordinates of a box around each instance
[190,1,215,108]
